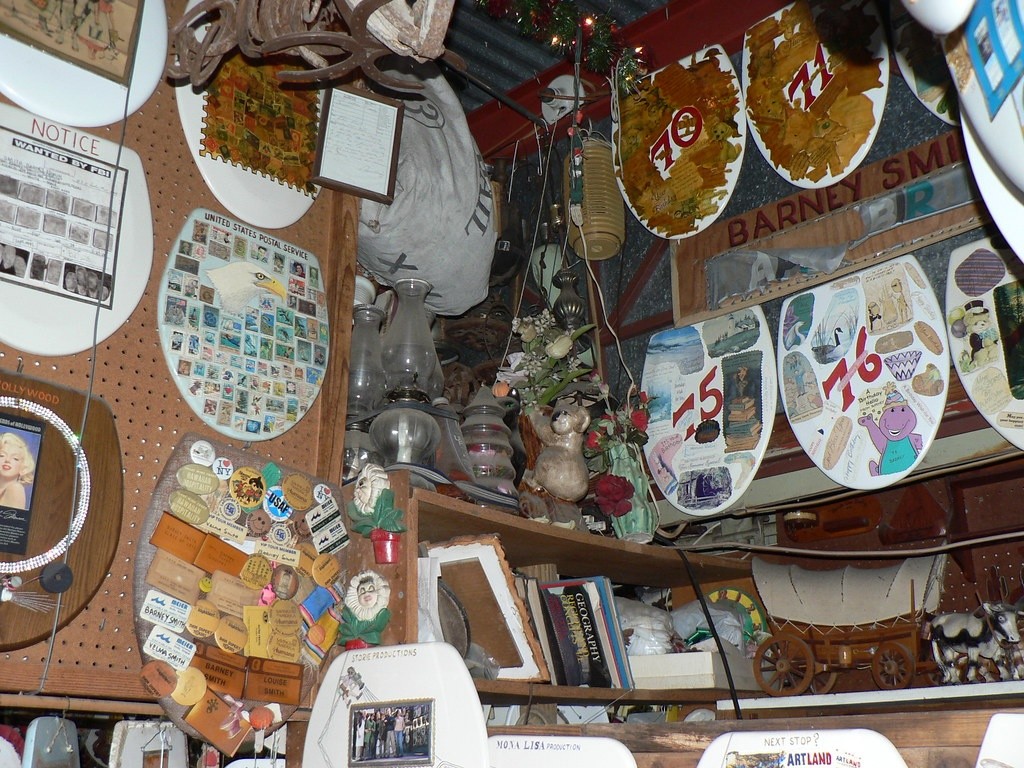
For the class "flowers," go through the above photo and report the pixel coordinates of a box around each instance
[495,309,610,405]
[581,366,651,517]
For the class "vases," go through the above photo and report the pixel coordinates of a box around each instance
[517,398,592,535]
[608,444,656,544]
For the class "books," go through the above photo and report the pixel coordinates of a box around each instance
[504,573,784,691]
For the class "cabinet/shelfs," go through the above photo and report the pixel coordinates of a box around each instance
[313,469,773,700]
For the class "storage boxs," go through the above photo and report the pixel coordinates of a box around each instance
[630,652,779,690]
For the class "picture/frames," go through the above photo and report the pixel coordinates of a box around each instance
[311,84,406,205]
[421,532,550,684]
[0,0,146,86]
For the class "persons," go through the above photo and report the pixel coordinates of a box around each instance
[0,433,35,511]
[354,707,406,761]
